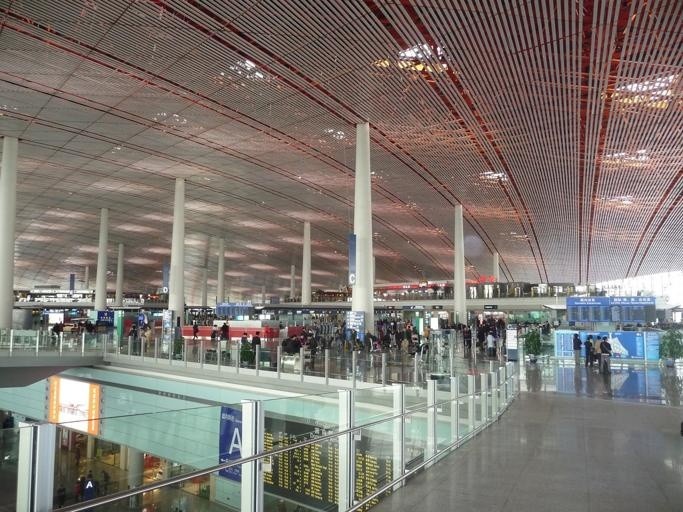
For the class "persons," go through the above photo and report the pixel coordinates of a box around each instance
[585,334,613,375]
[16,281,552,370]
[574,333,583,366]
[56,441,111,507]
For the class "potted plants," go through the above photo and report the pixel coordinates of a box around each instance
[519,330,542,361]
[240,341,254,368]
[173,336,185,360]
[659,329,683,367]
